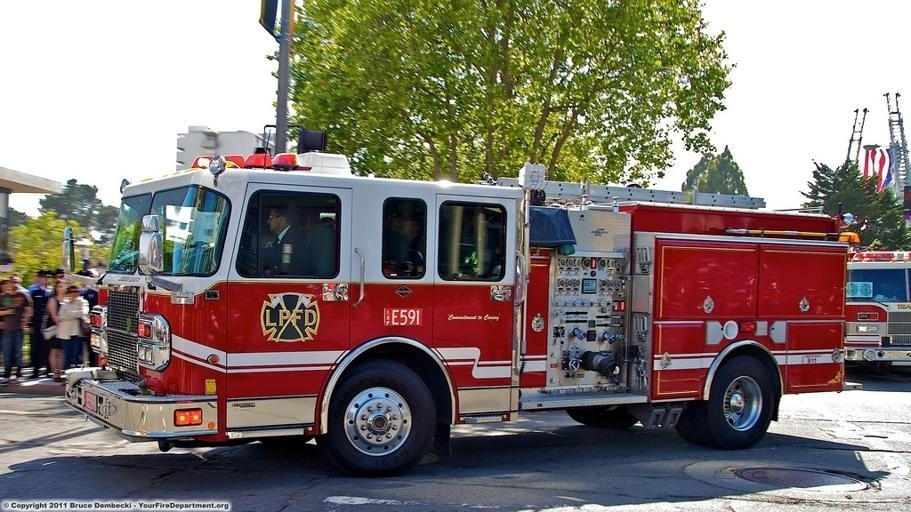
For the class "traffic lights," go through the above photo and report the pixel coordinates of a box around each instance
[296,126,326,151]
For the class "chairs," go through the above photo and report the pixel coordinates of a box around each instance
[315,224,337,278]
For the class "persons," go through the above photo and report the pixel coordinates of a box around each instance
[265,205,313,274]
[396,220,425,276]
[0,260,107,386]
[320,217,336,224]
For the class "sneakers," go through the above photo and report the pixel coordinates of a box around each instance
[0,375,64,386]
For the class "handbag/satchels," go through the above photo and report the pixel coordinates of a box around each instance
[80,317,91,334]
[41,324,57,340]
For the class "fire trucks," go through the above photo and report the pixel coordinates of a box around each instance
[61,153,911,476]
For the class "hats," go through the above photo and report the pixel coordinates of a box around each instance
[32,268,94,279]
